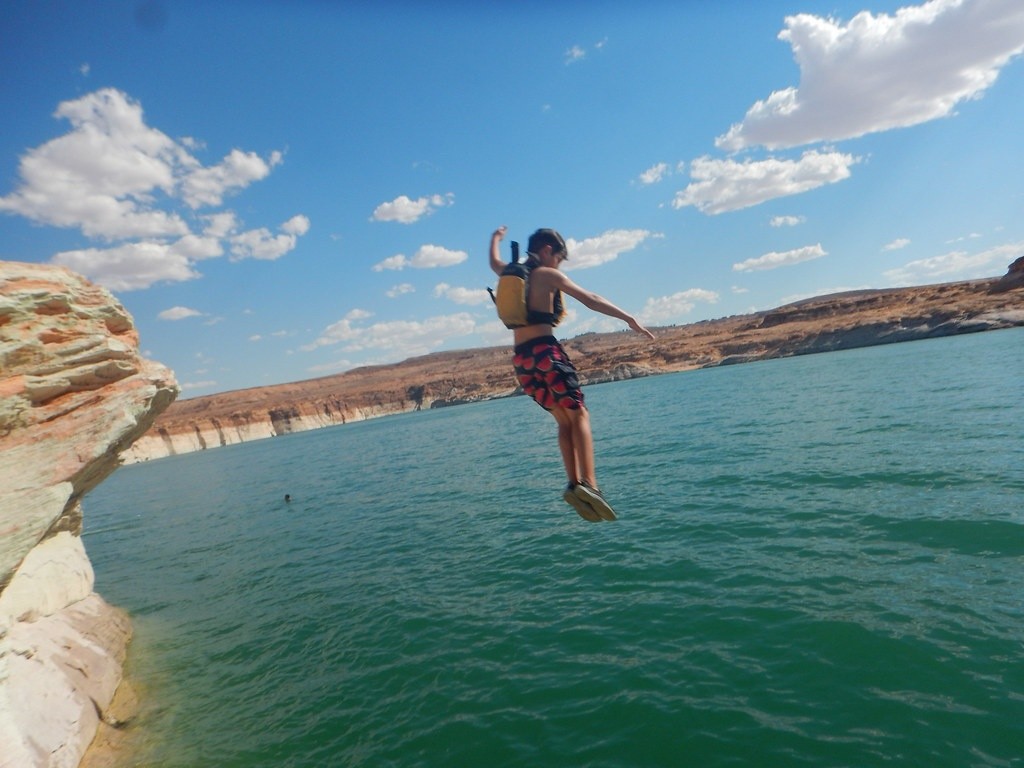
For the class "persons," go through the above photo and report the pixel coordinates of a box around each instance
[285,494,291,502]
[490,225,655,523]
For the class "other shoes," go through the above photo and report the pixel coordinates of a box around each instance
[564,484,602,522]
[574,482,616,521]
